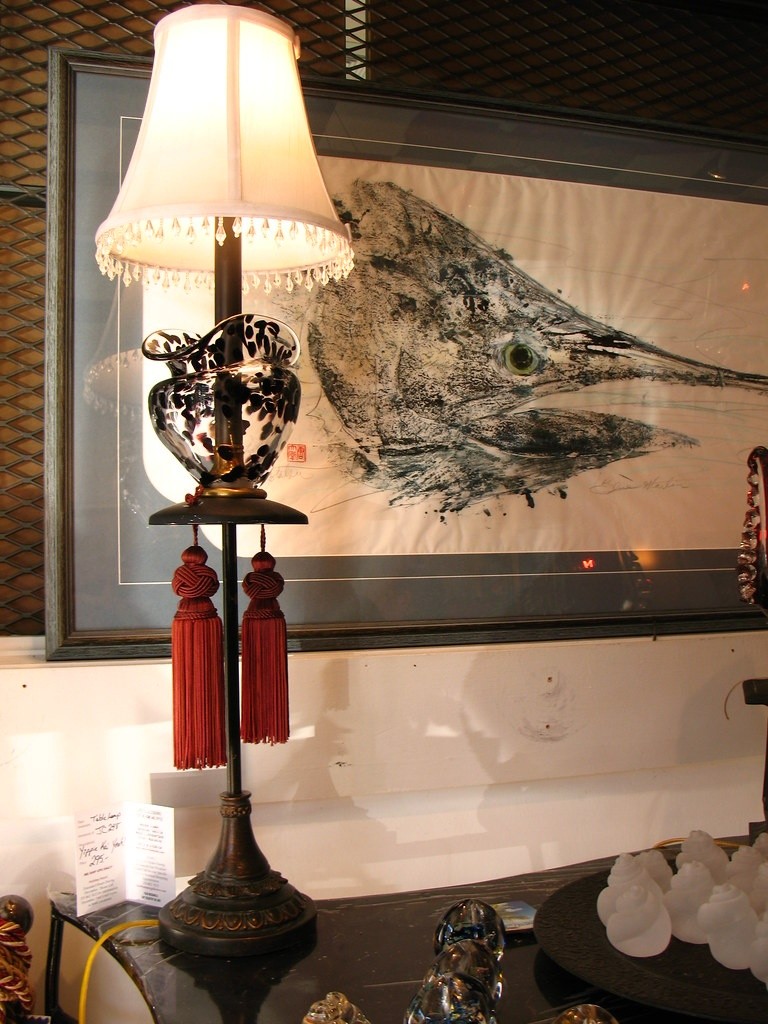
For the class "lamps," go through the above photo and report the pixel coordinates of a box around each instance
[88,4,365,964]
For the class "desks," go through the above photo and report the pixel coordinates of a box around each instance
[39,825,768,1024]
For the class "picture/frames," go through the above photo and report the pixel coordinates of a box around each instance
[42,47,768,660]
